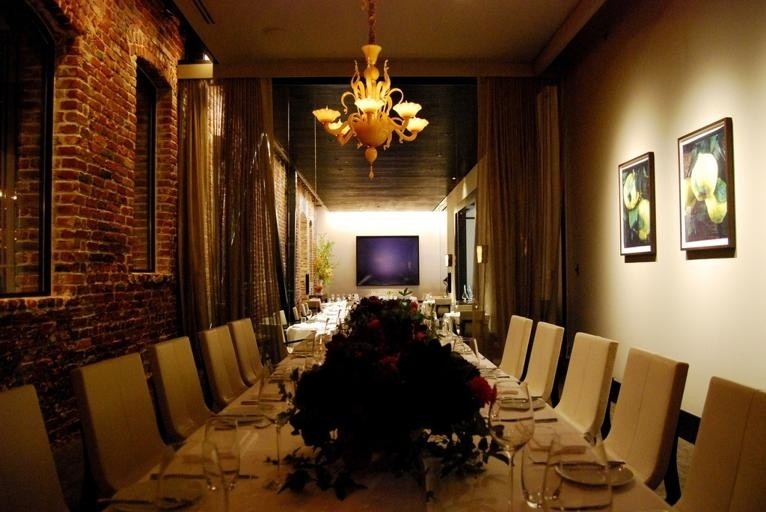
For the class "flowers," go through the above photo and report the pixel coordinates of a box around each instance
[260,297,510,498]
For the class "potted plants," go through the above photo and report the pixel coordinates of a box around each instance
[313,231,335,294]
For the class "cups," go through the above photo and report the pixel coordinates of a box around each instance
[452,337,480,372]
[520,424,612,512]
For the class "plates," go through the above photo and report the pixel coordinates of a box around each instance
[499,398,544,411]
[111,479,202,509]
[557,459,633,488]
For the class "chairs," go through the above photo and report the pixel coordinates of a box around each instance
[1,383,70,512]
[226,318,271,387]
[499,314,533,381]
[275,291,488,364]
[198,325,248,407]
[70,351,173,495]
[521,321,565,402]
[146,336,213,444]
[603,348,689,504]
[553,331,618,443]
[672,376,766,512]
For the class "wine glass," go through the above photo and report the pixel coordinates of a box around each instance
[489,378,535,509]
[300,291,357,328]
[156,330,325,512]
[388,293,452,341]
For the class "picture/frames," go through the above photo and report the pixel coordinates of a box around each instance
[618,151,657,257]
[676,117,738,251]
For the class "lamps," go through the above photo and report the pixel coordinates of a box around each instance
[310,22,430,181]
[445,253,453,266]
[476,244,489,264]
